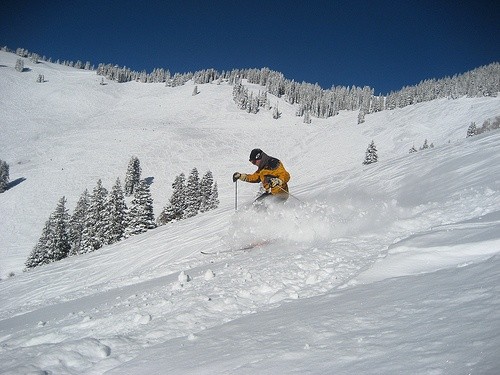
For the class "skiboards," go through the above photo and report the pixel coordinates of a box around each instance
[201,241,268,255]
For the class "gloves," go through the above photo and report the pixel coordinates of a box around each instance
[233,172,241,182]
[269,177,282,189]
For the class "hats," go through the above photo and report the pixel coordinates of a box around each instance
[249,149,262,161]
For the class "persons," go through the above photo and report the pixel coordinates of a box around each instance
[233,149,291,212]
